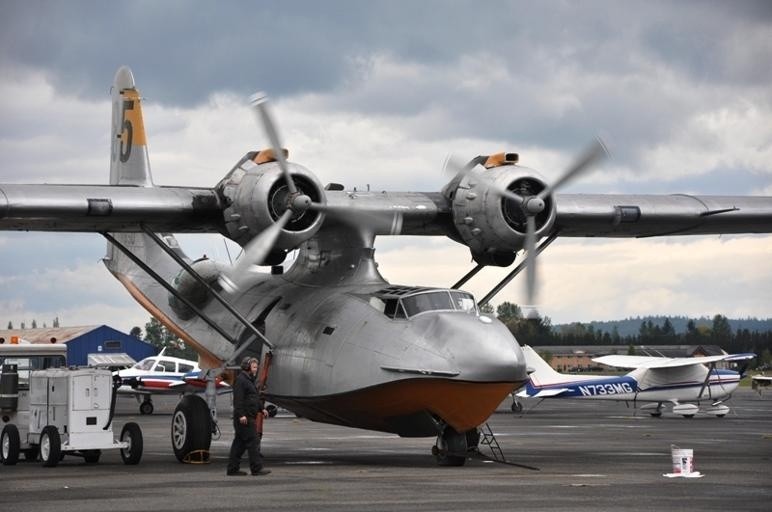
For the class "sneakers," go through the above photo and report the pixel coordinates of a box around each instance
[251,469,272,476]
[227,469,248,476]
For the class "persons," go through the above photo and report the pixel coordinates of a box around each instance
[226,355,273,477]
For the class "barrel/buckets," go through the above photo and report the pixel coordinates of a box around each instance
[671,443,693,473]
